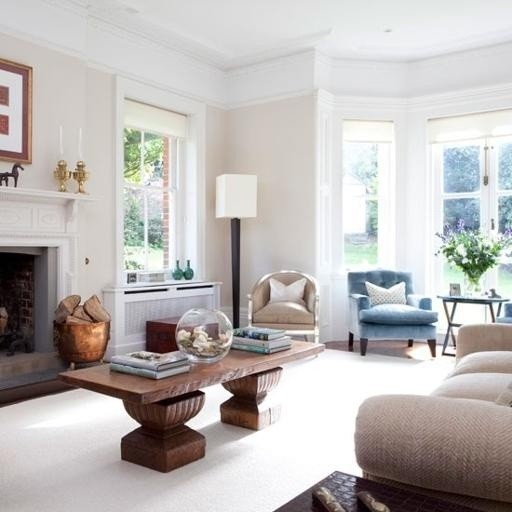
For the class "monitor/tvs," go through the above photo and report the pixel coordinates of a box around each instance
[230,327,295,354]
[113,350,192,378]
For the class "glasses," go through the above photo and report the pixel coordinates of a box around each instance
[215,172,258,329]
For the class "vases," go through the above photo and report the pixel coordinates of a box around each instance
[436,219,510,279]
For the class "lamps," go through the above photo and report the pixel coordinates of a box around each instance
[247,269,320,359]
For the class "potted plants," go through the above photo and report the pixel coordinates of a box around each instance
[0,58,35,164]
[127,273,138,285]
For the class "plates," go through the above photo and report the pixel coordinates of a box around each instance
[0,191,97,377]
[117,280,222,341]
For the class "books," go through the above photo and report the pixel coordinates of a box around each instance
[147,316,181,352]
[138,272,166,282]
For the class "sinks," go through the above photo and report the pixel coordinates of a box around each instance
[355,322,511,502]
[346,271,439,359]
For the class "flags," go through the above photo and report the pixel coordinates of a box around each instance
[468,277,484,297]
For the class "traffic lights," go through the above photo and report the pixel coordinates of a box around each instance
[268,277,307,305]
[365,278,407,306]
